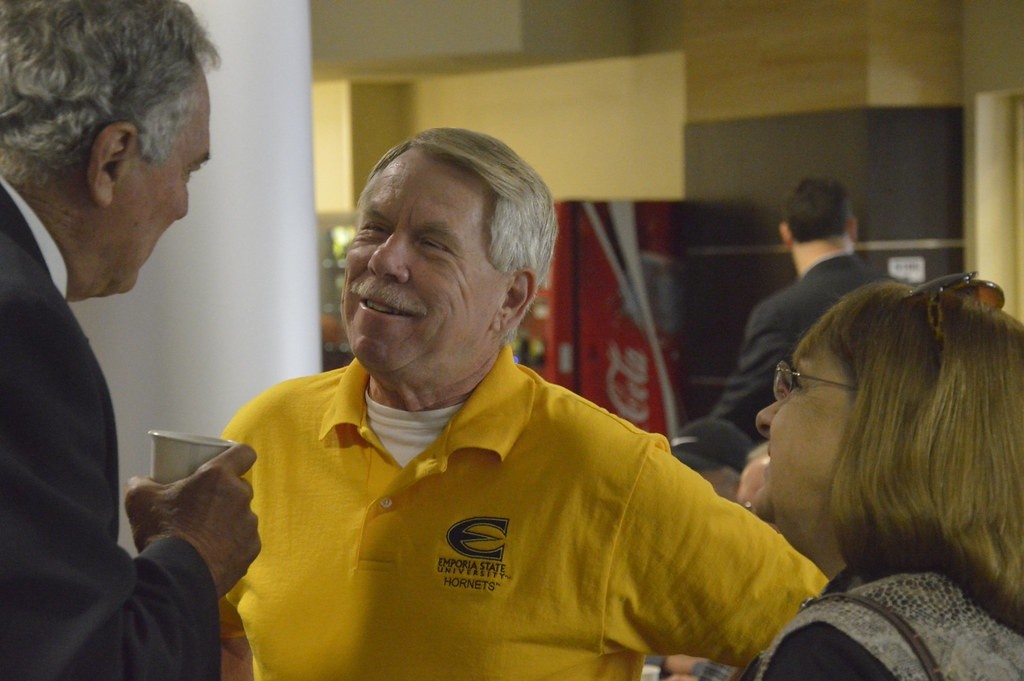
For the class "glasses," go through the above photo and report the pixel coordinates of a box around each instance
[772,361,857,402]
[900,271,1005,376]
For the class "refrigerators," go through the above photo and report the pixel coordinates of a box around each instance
[508,200,693,442]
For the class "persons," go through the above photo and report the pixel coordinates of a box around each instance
[750,280,1024,681]
[663,418,776,681]
[217,128,829,681]
[0,0,264,681]
[708,178,890,443]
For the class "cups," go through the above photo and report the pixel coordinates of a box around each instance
[148,430,235,483]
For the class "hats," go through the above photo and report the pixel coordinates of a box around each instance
[669,417,753,474]
